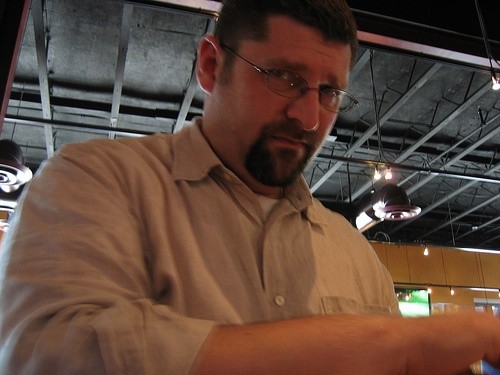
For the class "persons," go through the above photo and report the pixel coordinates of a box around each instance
[0,0,500,375]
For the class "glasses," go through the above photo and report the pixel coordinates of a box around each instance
[216,41,358,113]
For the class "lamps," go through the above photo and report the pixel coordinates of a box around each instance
[369,49,393,180]
[373,185,422,221]
[0,92,33,186]
[475,0,500,91]
[355,205,382,233]
